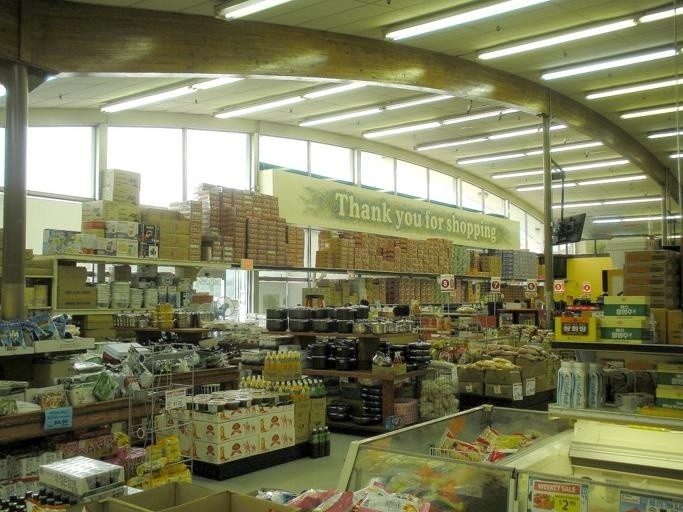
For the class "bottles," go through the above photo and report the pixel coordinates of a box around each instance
[240,350,327,402]
[310,428,319,458]
[556,360,607,409]
[318,427,324,456]
[324,427,331,456]
[0,488,78,511]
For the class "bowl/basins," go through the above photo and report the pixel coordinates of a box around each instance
[97,280,158,309]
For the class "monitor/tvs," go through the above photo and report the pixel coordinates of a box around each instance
[552,212,587,246]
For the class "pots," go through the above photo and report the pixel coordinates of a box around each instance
[378,340,431,371]
[327,385,382,425]
[307,338,358,371]
[266,303,414,334]
[113,303,215,328]
